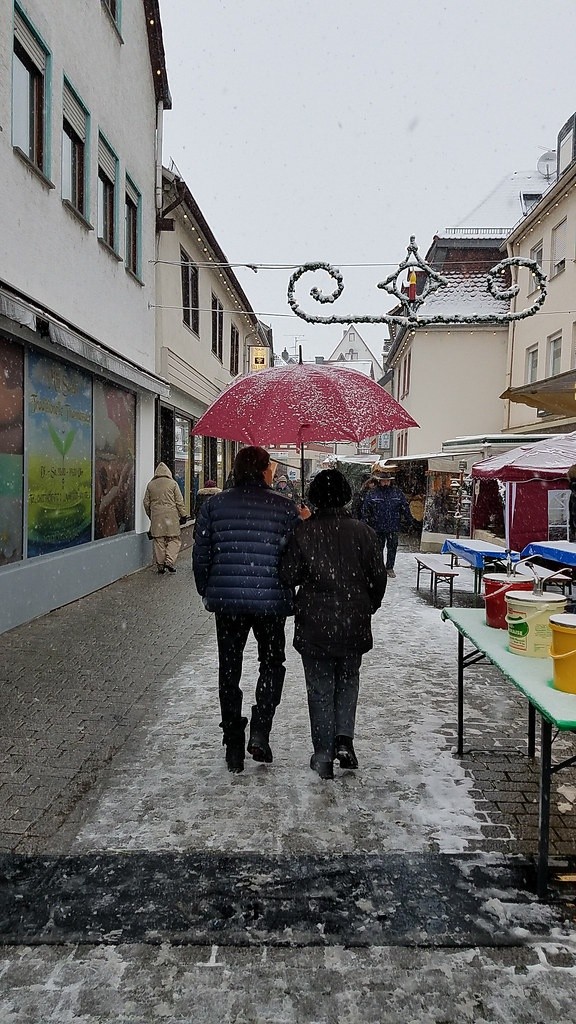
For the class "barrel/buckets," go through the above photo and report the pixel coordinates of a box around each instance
[482,577,535,630]
[548,620,576,694]
[503,596,568,658]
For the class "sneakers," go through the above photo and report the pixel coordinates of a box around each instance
[310,754,334,780]
[336,735,358,770]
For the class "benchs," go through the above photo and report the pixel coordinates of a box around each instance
[414,556,459,608]
[494,559,572,595]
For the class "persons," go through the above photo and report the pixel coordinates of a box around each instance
[568,464,576,542]
[143,462,186,573]
[192,447,312,772]
[195,468,417,577]
[277,468,387,780]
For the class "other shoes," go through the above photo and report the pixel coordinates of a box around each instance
[386,569,396,578]
[158,562,176,574]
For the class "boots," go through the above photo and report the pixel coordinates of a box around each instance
[219,716,248,773]
[247,706,273,763]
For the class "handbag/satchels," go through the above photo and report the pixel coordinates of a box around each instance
[147,526,153,540]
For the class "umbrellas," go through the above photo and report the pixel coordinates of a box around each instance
[190,344,421,507]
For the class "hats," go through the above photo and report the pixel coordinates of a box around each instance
[234,446,270,473]
[276,476,287,484]
[205,480,216,488]
[361,473,373,484]
[307,468,351,508]
[373,472,395,480]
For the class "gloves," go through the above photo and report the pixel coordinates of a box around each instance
[179,517,187,525]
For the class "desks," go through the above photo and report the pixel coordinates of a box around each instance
[441,608,576,878]
[521,540,576,586]
[440,538,521,594]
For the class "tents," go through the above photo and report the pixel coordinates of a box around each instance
[323,369,576,550]
